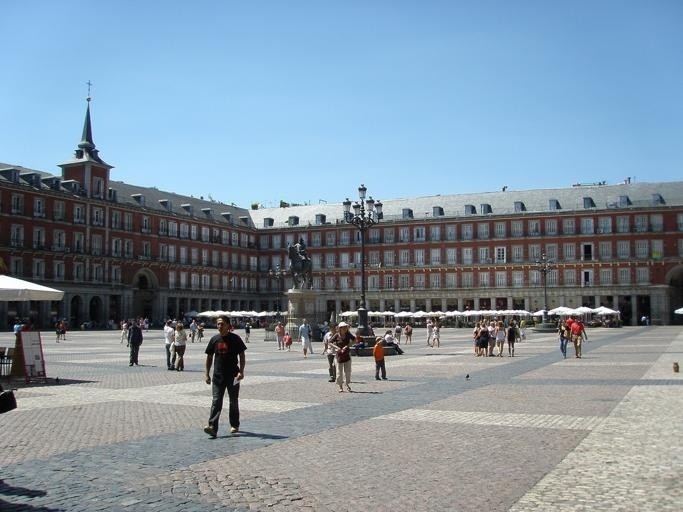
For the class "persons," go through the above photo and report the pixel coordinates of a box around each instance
[8,312,204,370]
[641,314,650,325]
[329,320,356,394]
[244,315,526,359]
[204,315,247,437]
[372,336,388,380]
[295,234,307,266]
[321,322,337,383]
[554,315,588,360]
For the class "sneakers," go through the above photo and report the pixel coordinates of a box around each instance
[328,377,387,392]
[204,426,217,437]
[230,427,238,433]
[489,352,511,357]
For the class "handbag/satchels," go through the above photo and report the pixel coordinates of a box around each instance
[336,350,348,363]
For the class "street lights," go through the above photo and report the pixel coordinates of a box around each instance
[342,183,384,337]
[534,252,554,324]
[268,263,286,326]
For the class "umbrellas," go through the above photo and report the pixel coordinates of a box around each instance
[339,306,622,328]
[0,274,66,302]
[674,307,683,314]
[184,309,288,319]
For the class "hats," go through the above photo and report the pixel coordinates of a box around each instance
[338,322,348,327]
[216,315,232,329]
[376,337,384,344]
